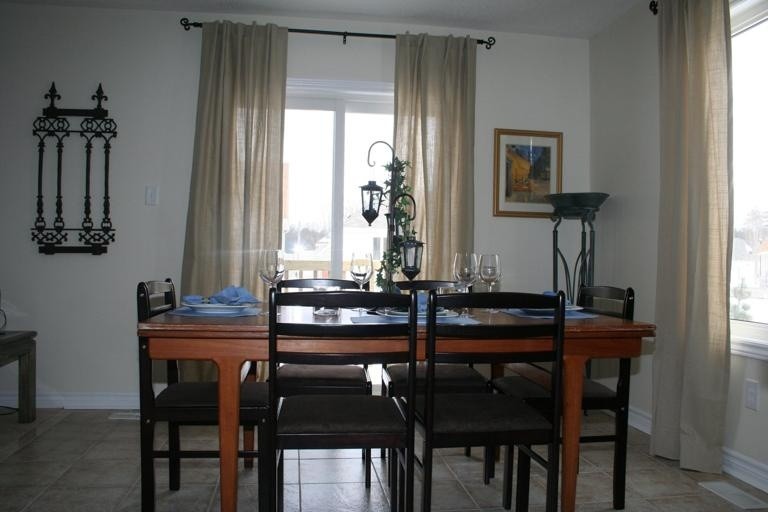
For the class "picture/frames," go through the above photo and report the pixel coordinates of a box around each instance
[492,128,564,219]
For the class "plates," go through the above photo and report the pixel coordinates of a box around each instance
[520,305,584,314]
[184,305,249,314]
[375,308,459,319]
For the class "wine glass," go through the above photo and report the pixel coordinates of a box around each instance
[350,252,373,312]
[257,250,285,316]
[454,253,500,316]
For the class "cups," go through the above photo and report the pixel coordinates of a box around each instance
[313,269,342,316]
[436,286,469,320]
